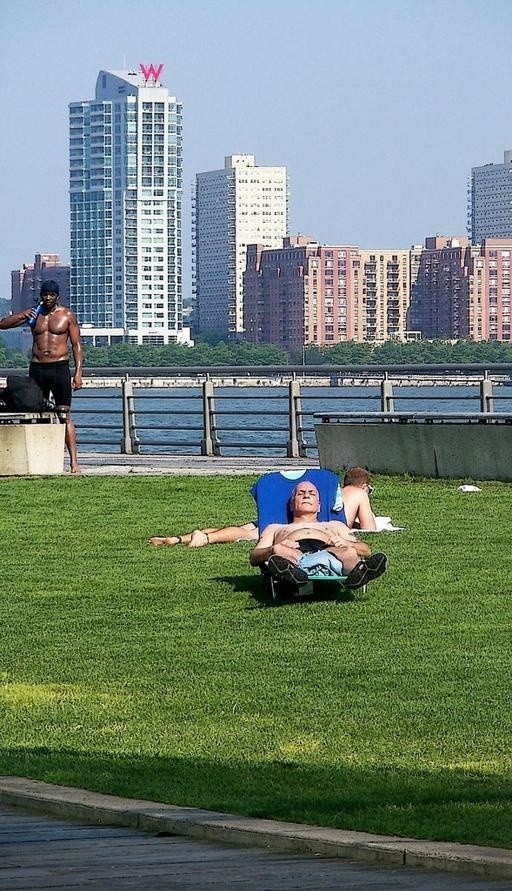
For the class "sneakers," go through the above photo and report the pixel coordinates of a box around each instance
[267,554,308,587]
[347,553,387,590]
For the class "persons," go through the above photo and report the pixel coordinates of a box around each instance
[248,481,389,592]
[0,278,86,474]
[145,465,378,549]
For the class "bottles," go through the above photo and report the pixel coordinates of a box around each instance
[27,301,44,327]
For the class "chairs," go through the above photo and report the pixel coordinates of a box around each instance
[255,468,353,604]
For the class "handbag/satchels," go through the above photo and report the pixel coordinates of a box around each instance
[6,375,44,412]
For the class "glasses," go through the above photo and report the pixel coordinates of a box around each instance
[368,484,374,495]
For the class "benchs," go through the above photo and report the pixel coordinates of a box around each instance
[314,413,510,481]
[0,412,68,480]
